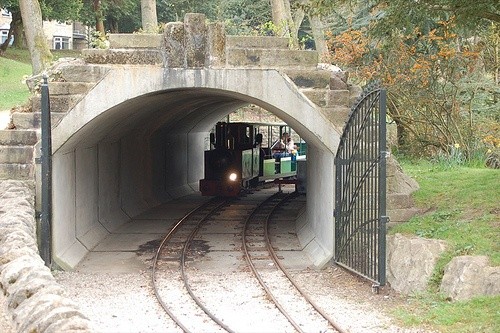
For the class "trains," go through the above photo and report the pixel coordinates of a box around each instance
[198,120,308,199]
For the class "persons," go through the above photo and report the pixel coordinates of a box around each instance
[274,132,299,160]
